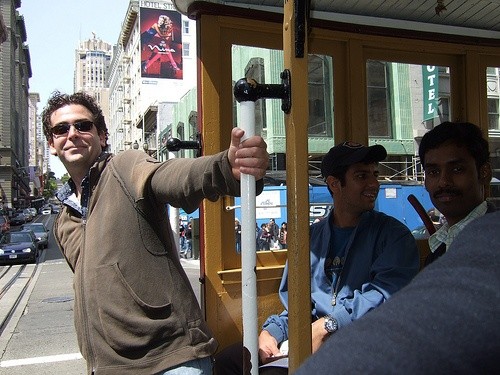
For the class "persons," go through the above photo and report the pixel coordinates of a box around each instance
[418,121,500,264]
[176,216,195,259]
[235,220,241,254]
[41,90,273,375]
[255,218,288,251]
[292,207,500,375]
[211,140,422,375]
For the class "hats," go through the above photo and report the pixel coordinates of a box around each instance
[321,141,388,178]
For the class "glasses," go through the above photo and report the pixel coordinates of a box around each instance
[49,121,97,135]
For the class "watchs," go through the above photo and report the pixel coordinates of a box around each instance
[323,314,338,334]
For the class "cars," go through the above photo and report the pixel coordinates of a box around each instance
[0,208,37,231]
[19,223,50,247]
[0,230,40,264]
[42,208,52,215]
